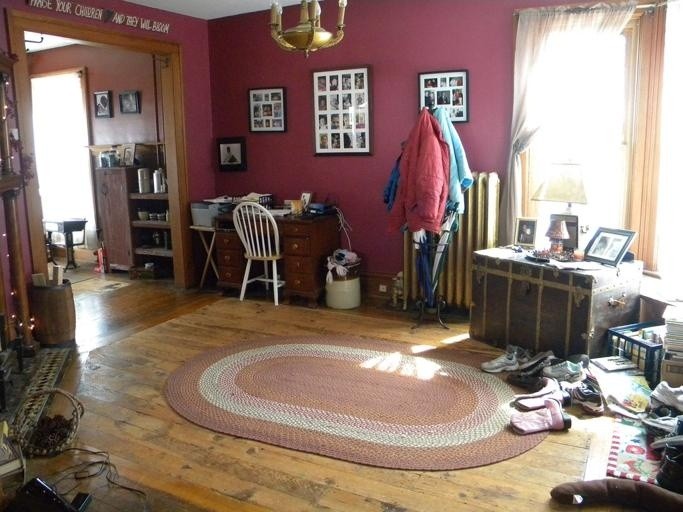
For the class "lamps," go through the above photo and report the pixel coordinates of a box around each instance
[531,163,588,214]
[270,0,347,59]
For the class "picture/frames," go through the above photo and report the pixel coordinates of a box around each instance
[514,217,537,248]
[417,69,470,124]
[247,86,287,133]
[119,143,135,166]
[118,90,139,113]
[216,136,246,172]
[584,228,636,266]
[92,90,113,118]
[311,65,374,155]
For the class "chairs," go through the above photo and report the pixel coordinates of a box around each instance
[232,202,285,306]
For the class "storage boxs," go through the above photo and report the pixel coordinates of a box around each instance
[660,352,683,388]
[469,245,643,360]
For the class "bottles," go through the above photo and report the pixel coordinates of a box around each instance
[110,145,121,168]
[155,232,160,245]
[137,167,165,193]
[100,151,110,168]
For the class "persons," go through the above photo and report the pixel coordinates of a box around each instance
[316,73,366,150]
[423,76,463,118]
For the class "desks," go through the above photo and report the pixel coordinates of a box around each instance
[190,226,219,288]
[41,220,86,273]
[213,210,340,309]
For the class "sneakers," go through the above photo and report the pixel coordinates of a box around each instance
[518,349,555,376]
[559,379,604,416]
[542,360,586,382]
[480,352,519,372]
[505,344,532,363]
[638,403,682,450]
[649,380,683,412]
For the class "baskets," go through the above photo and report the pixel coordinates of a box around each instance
[606,319,664,390]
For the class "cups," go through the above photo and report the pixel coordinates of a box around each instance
[291,200,303,214]
[136,208,169,222]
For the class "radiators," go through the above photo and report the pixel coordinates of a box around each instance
[401,171,500,310]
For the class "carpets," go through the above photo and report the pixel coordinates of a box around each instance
[165,334,552,471]
[0,344,74,453]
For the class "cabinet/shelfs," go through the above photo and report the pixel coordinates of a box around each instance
[93,166,173,273]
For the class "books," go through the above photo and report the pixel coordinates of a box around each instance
[586,318,683,420]
[232,190,336,217]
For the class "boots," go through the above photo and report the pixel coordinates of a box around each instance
[512,374,571,411]
[510,398,571,435]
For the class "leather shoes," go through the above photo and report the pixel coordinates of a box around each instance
[660,443,682,466]
[507,374,539,388]
[656,455,683,491]
